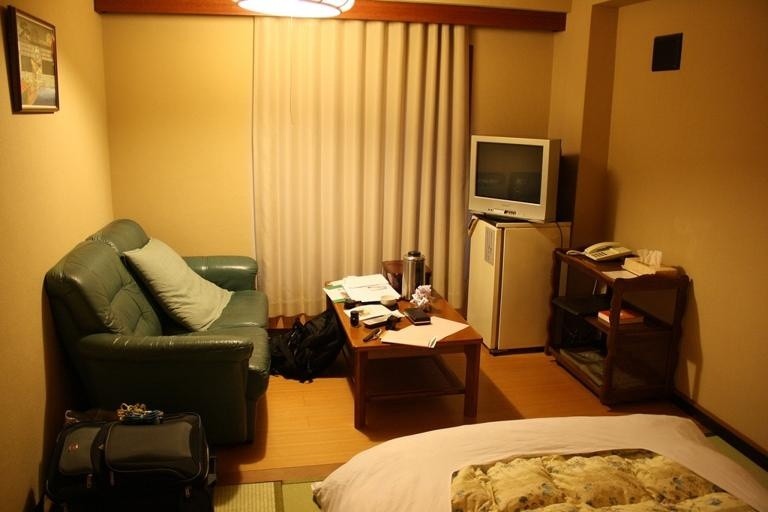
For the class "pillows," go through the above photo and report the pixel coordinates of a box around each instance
[123,236,233,334]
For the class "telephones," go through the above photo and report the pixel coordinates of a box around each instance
[584,241,632,261]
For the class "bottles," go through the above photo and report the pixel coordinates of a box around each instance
[401,250,425,301]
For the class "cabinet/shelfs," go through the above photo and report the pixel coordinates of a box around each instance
[547,247,690,409]
[466,214,574,358]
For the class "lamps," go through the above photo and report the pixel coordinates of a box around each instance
[232,0,355,19]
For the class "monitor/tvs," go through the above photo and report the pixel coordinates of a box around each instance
[469,134,562,223]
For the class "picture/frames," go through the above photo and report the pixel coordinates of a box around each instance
[3,4,61,114]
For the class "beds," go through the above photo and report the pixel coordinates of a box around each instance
[314,409,768,512]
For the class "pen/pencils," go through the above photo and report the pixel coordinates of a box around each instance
[363,328,380,341]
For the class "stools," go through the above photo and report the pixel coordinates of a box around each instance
[382,259,432,292]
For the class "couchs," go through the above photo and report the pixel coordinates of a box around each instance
[44,220,274,449]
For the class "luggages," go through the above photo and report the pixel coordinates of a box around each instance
[46,411,215,504]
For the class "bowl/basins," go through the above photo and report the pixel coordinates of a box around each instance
[380,295,396,307]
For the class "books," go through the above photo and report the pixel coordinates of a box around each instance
[598,308,644,324]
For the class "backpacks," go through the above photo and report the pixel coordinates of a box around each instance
[270,305,343,379]
